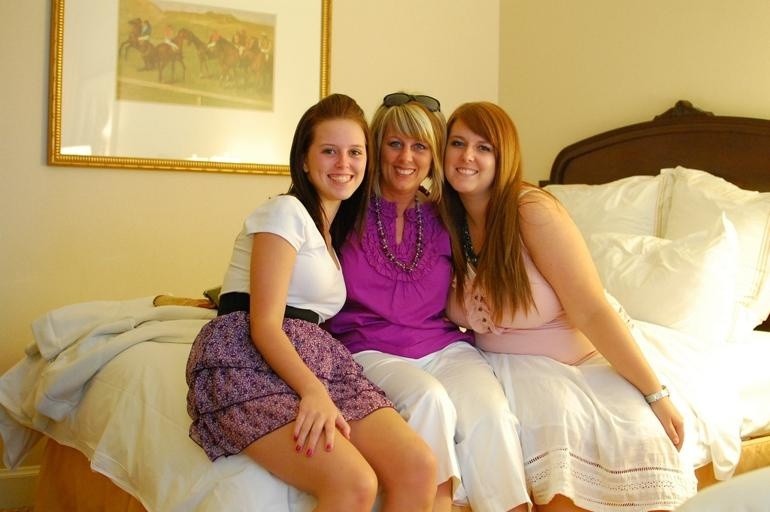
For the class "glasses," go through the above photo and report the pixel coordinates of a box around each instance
[382,92,443,113]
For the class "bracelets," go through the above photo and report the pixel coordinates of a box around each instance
[645,385,671,403]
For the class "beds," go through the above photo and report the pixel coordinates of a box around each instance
[39,101,770,512]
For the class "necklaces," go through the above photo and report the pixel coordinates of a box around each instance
[372,190,426,272]
[460,216,484,268]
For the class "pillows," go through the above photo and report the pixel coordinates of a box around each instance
[543,166,767,340]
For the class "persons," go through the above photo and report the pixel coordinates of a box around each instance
[186,95,440,512]
[321,91,537,512]
[436,100,699,511]
[136,19,273,65]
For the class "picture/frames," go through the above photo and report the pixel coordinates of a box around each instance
[46,1,332,180]
